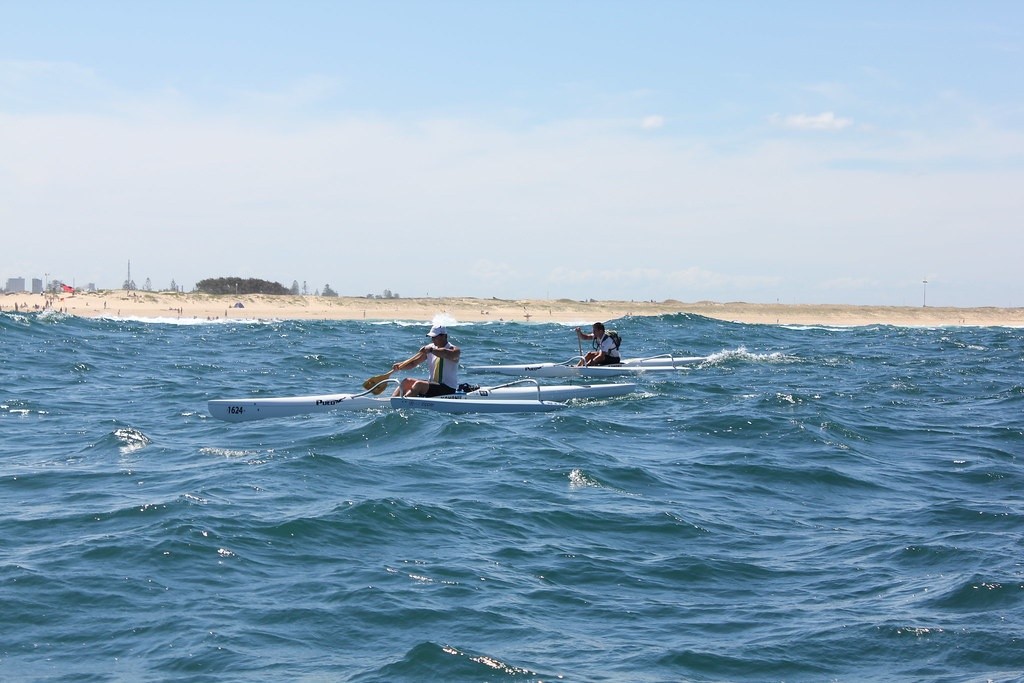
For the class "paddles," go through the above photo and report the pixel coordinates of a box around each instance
[576,327,582,359]
[363,347,427,395]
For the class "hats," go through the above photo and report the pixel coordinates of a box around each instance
[426,324,447,338]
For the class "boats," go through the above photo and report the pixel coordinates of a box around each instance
[467,353,709,378]
[206,377,637,425]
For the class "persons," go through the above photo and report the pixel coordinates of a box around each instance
[390,324,460,398]
[575,322,621,367]
[15,292,964,324]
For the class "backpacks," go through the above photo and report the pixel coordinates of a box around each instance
[594,329,622,352]
